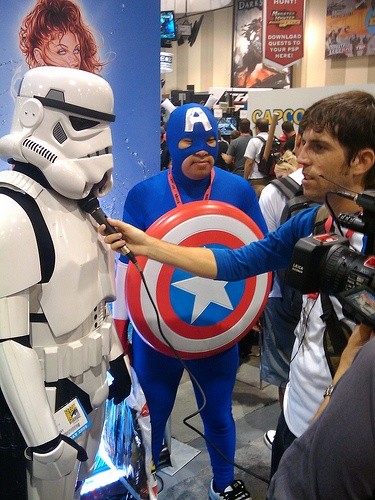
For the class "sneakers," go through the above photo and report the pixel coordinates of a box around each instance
[264,429,277,450]
[208,477,253,500]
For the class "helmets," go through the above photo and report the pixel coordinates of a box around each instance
[0,66,116,200]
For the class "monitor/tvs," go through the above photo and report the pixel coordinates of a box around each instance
[216,116,239,135]
[187,15,204,47]
[161,10,177,41]
[170,90,214,107]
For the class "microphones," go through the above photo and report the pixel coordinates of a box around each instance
[79,193,140,271]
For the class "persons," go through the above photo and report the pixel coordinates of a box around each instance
[0,66,134,500]
[18,0,111,77]
[116,103,276,500]
[99,91,375,500]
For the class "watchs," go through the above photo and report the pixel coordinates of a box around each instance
[323,384,334,397]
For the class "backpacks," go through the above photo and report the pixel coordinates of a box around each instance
[270,175,323,317]
[254,136,280,176]
